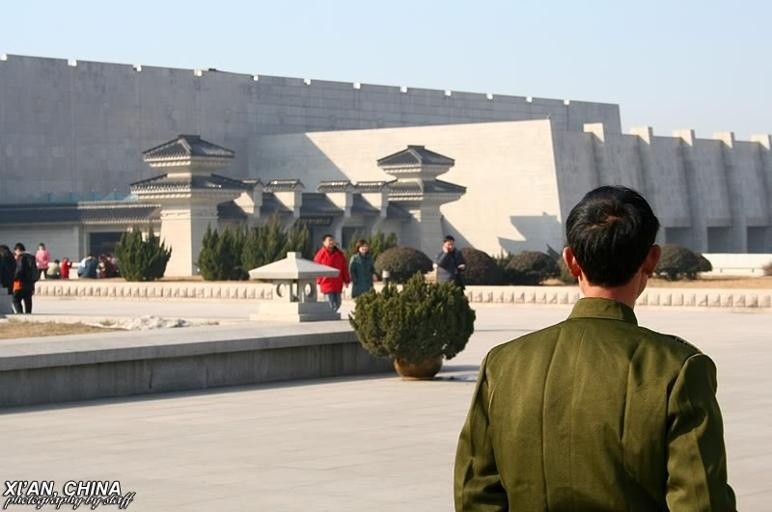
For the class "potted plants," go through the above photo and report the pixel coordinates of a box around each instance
[347,271,477,382]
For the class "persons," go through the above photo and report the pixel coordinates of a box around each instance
[0,241,119,314]
[454,183,738,512]
[348,239,378,299]
[435,235,466,291]
[313,235,351,311]
